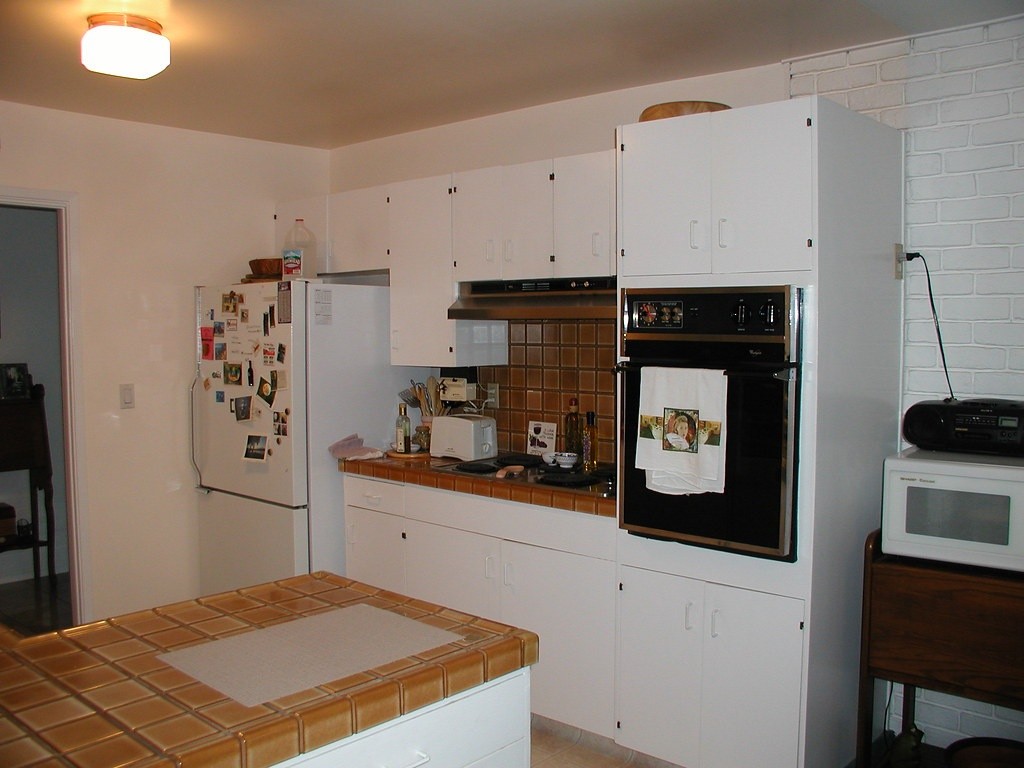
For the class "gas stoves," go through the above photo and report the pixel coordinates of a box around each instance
[438,453,615,489]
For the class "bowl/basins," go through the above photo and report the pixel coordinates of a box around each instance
[555,453,578,468]
[542,453,563,466]
[249,258,282,275]
[410,444,420,453]
[390,443,397,451]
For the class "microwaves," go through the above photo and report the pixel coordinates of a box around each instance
[881,446,1024,572]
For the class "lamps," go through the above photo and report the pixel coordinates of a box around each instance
[78,13,170,79]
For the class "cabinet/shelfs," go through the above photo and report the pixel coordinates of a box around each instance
[341,472,1024,768]
[0,400,56,594]
[274,94,906,372]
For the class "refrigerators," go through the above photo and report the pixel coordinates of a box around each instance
[188,283,441,597]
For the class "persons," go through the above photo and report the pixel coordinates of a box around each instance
[656,417,662,426]
[672,415,688,438]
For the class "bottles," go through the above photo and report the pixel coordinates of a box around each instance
[412,426,430,451]
[283,220,317,283]
[396,403,410,453]
[566,398,582,465]
[583,412,598,471]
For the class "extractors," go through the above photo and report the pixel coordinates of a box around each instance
[448,276,616,319]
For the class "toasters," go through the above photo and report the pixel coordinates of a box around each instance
[430,415,498,461]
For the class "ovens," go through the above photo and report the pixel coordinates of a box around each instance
[614,287,798,564]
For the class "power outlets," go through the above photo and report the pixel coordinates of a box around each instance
[894,243,904,281]
[487,383,500,409]
